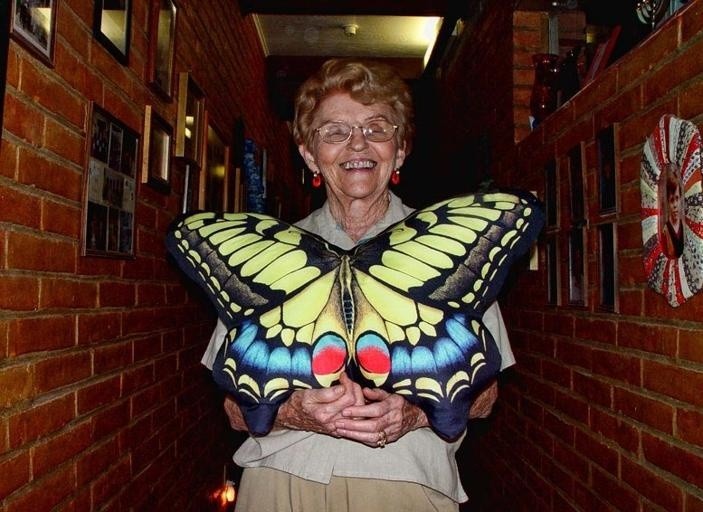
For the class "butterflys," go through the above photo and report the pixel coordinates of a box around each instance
[164,187,547,444]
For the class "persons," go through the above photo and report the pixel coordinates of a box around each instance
[657,175,687,260]
[196,52,519,512]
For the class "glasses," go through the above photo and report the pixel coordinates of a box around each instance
[301,119,402,149]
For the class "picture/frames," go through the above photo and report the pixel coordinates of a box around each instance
[174,70,247,218]
[80,101,141,260]
[9,1,59,68]
[92,1,132,67]
[147,2,177,102]
[141,104,173,195]
[541,111,702,315]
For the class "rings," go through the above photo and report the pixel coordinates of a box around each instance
[378,430,386,440]
[376,439,386,448]
